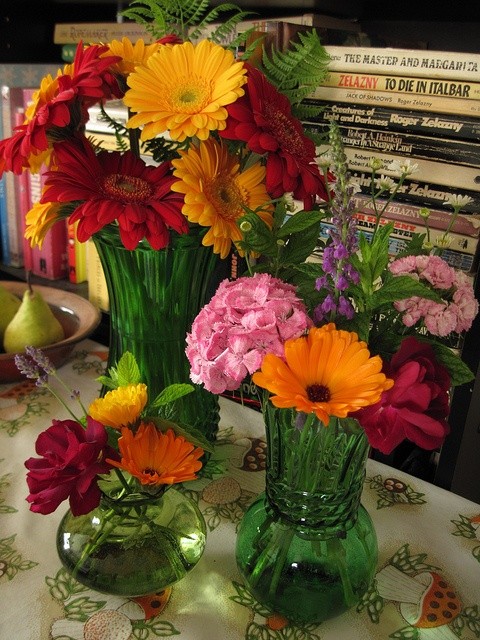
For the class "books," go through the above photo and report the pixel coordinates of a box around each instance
[293,46,480,465]
[0,15,365,413]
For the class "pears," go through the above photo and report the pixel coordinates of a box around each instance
[0,286,22,351]
[4,269,64,354]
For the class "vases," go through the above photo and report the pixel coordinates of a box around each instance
[55,469,209,597]
[81,219,230,478]
[235,390,377,624]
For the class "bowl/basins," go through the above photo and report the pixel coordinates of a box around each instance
[0,281,103,383]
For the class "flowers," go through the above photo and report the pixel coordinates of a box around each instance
[0,0,338,407]
[9,344,218,577]
[182,116,478,605]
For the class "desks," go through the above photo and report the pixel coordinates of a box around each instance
[0,347,480,640]
